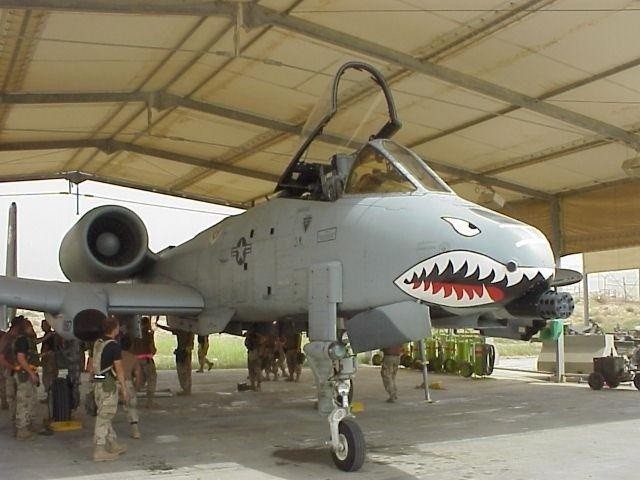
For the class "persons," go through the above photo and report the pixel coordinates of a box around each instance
[156,324,193,395]
[196,335,214,372]
[1,315,59,438]
[244,320,301,389]
[377,340,403,404]
[86,317,129,460]
[120,317,157,406]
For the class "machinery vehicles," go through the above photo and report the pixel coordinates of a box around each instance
[586,347,640,391]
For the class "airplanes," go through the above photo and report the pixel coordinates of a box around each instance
[1,57,583,473]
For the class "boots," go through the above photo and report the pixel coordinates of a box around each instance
[196,365,204,372]
[109,441,127,454]
[31,423,54,435]
[131,424,140,439]
[247,369,302,392]
[207,362,214,371]
[93,444,120,462]
[17,427,37,441]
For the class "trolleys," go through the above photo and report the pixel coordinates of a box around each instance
[370,323,499,376]
